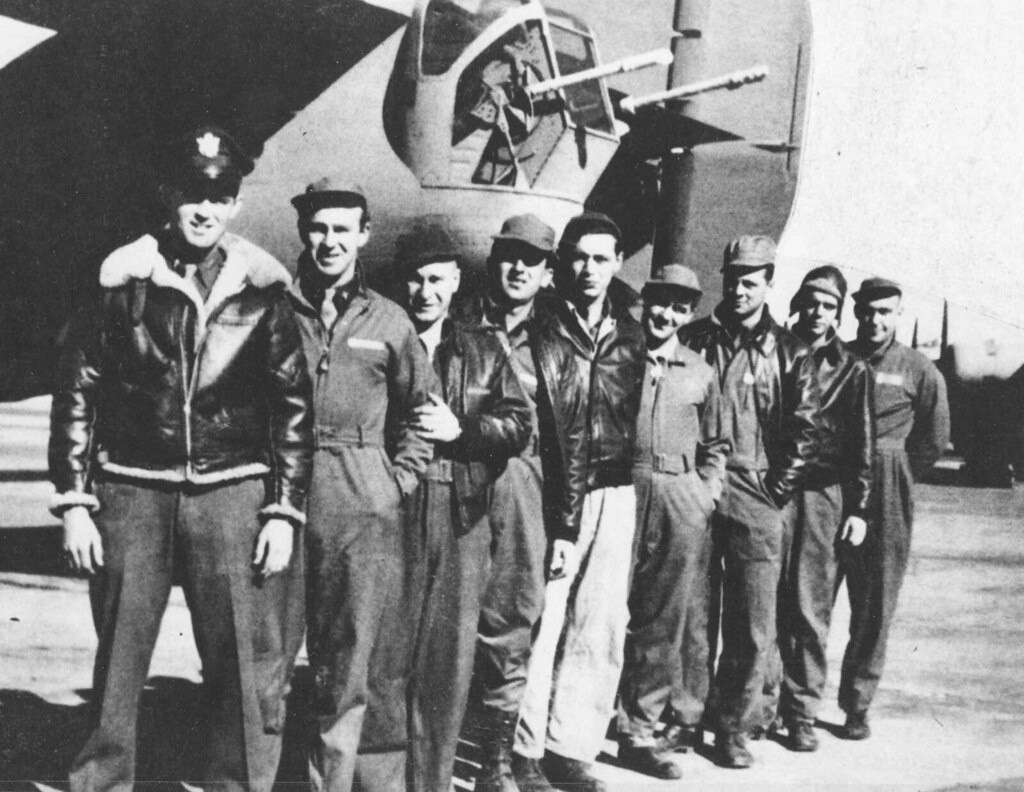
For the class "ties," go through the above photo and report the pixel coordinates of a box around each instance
[183,263,197,285]
[316,289,338,335]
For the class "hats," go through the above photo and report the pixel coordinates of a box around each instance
[850,277,902,303]
[388,212,462,279]
[492,213,555,253]
[290,175,366,211]
[790,266,847,328]
[559,213,622,259]
[644,265,703,306]
[161,127,256,198]
[721,235,776,283]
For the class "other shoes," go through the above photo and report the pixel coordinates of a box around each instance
[618,743,682,780]
[844,710,870,738]
[694,725,752,768]
[753,719,778,740]
[473,764,520,792]
[540,749,605,792]
[788,719,819,750]
[652,722,705,754]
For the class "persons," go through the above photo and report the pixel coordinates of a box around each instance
[654,233,822,768]
[606,264,739,782]
[273,177,443,792]
[475,217,590,792]
[832,277,951,740]
[512,213,647,792]
[48,127,315,792]
[776,266,876,752]
[351,224,534,792]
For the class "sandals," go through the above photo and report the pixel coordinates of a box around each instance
[511,756,563,792]
